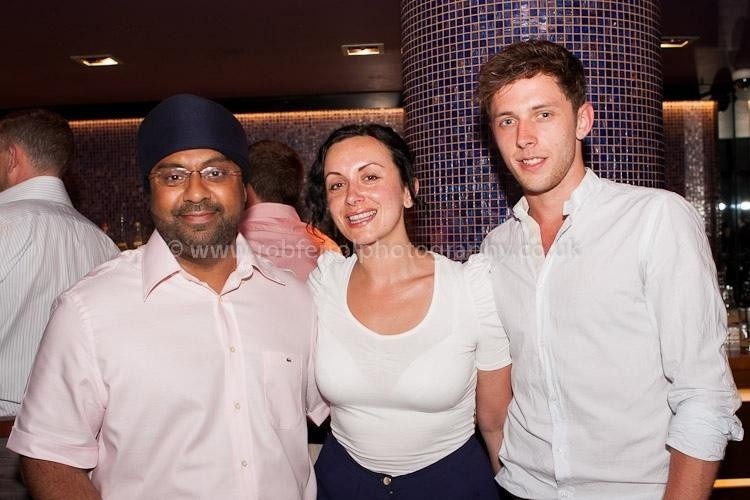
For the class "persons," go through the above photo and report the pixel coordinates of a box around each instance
[238,139,350,462]
[6,94,335,500]
[465,40,744,500]
[0,109,123,500]
[300,122,518,500]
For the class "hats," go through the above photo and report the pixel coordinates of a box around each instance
[136,93,250,176]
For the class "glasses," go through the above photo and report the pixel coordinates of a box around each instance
[151,166,242,187]
